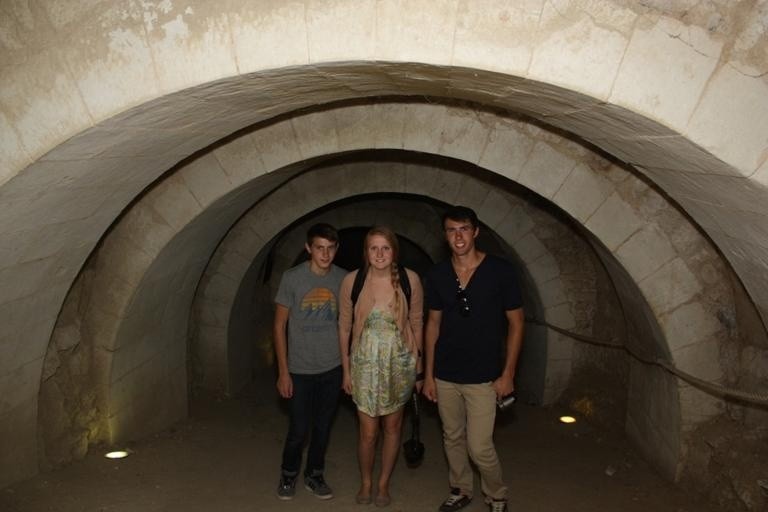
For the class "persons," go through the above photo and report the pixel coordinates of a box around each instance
[422,206,525,512]
[337,226,425,507]
[272,223,352,500]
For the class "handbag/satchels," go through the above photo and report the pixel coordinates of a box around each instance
[404,420,425,469]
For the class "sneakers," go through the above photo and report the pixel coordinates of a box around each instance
[489,498,508,512]
[303,475,334,500]
[277,477,297,500]
[439,487,471,512]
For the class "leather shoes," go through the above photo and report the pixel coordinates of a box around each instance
[375,492,390,508]
[356,488,372,504]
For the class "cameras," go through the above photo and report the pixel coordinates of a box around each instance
[497,395,515,409]
[403,439,424,468]
